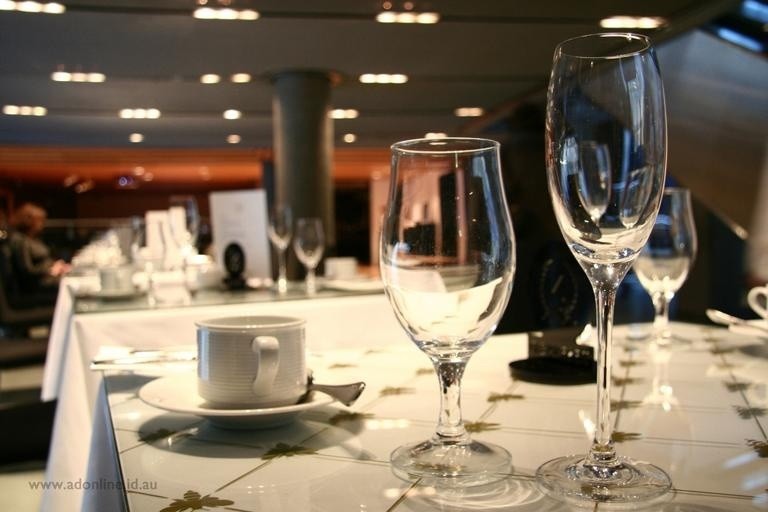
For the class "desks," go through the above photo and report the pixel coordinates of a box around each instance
[38,260,768,512]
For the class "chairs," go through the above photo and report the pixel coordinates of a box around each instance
[0,244,57,511]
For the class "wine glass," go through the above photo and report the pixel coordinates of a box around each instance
[628,188,699,352]
[536,31,672,507]
[168,197,199,267]
[379,137,519,486]
[268,204,325,297]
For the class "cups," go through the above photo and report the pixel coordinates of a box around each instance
[194,315,309,406]
[101,268,133,294]
[325,257,359,279]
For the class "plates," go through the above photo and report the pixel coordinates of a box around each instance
[140,374,334,431]
[728,319,768,341]
[90,289,146,300]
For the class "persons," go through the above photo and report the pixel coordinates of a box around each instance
[7,204,69,310]
